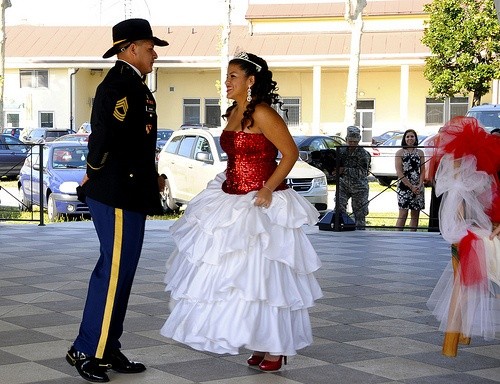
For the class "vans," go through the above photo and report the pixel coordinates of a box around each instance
[465,103,500,134]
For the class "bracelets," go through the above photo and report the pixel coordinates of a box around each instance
[409,184,413,188]
[264,185,273,193]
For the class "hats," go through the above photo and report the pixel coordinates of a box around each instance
[346,126,361,141]
[103,18,169,58]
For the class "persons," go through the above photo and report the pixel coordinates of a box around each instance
[333,126,371,230]
[491,127,500,136]
[160,49,325,372]
[395,129,425,232]
[65,18,170,383]
[489,224,500,241]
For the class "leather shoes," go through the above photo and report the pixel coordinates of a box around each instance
[99,347,146,372]
[65,346,109,382]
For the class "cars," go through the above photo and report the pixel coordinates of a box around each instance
[154,122,439,186]
[0,121,91,222]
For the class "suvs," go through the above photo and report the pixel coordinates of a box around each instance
[157,123,327,216]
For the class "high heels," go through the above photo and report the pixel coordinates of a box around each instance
[247,354,264,365]
[259,354,287,370]
